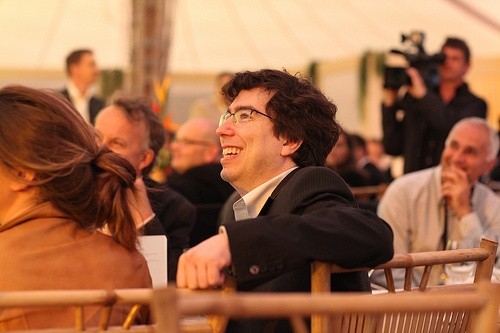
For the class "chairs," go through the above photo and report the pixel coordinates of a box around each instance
[154,283,500,333]
[310,236,500,292]
[0,279,235,333]
[350,184,387,209]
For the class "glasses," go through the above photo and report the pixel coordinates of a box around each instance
[218,107,293,129]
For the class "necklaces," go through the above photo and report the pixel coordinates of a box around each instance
[442,185,474,251]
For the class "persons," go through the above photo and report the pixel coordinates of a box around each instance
[94,94,213,287]
[381,36,489,174]
[369,117,500,290]
[176,69,395,333]
[170,117,500,250]
[60,49,106,127]
[0,84,153,333]
